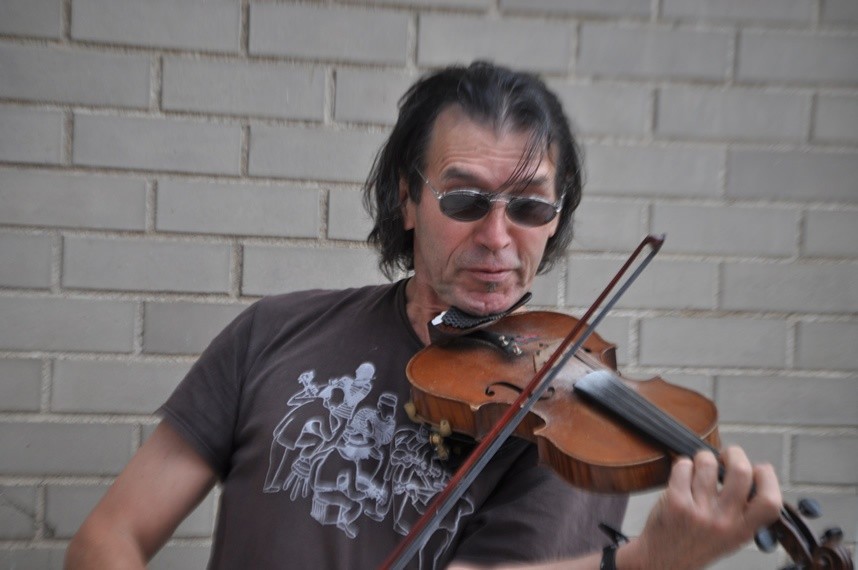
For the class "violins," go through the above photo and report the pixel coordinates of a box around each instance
[406,311,853,570]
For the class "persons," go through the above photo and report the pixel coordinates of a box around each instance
[59,60,785,570]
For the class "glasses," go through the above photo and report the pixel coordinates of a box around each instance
[415,164,567,229]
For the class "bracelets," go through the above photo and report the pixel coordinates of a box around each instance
[600,524,632,570]
